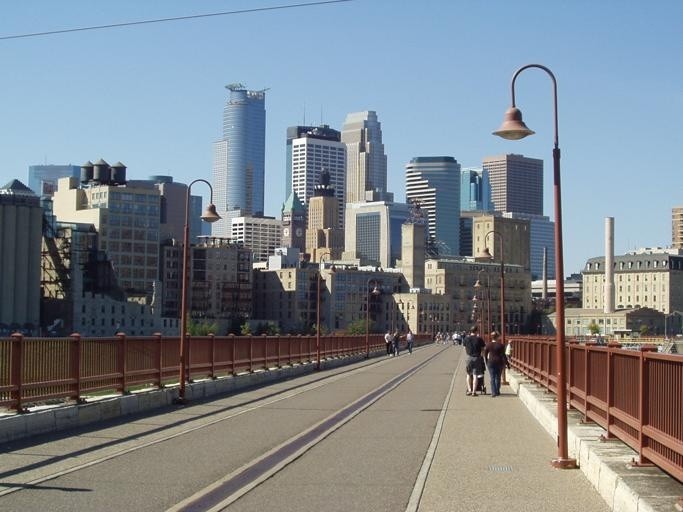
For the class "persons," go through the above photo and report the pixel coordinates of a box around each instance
[461,324,486,397]
[382,327,415,358]
[433,329,470,348]
[483,330,510,397]
[497,338,510,385]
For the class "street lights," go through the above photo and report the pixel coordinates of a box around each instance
[316,251,337,371]
[171,176,224,406]
[366,276,382,353]
[391,294,440,339]
[469,268,492,346]
[491,60,579,472]
[479,230,510,387]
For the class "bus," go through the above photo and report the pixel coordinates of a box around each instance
[612,329,632,337]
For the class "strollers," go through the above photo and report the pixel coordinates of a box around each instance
[466,356,487,395]
